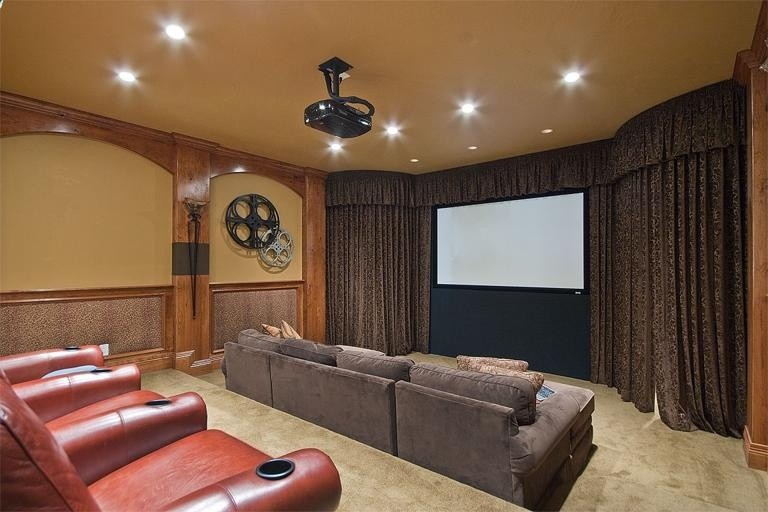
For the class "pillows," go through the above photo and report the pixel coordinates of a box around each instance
[260,323,280,338]
[456,354,529,372]
[477,363,544,394]
[280,320,301,340]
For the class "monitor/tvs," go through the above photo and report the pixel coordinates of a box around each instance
[433,189,588,293]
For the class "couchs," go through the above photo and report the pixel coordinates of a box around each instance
[222,329,595,509]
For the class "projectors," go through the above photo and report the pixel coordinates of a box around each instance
[303,100,373,139]
